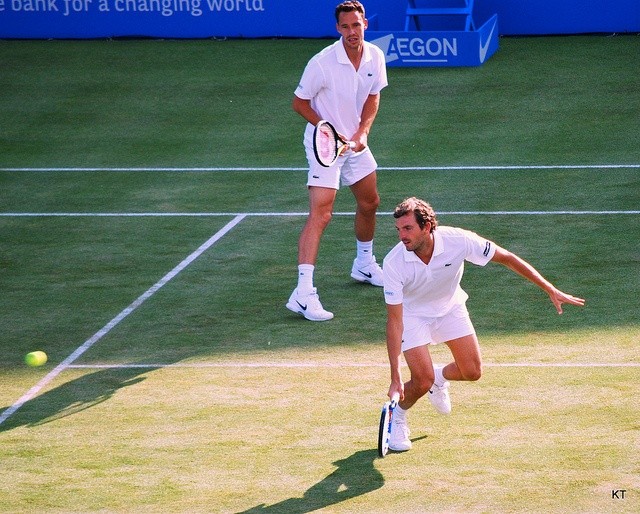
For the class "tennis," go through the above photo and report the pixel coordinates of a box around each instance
[25,351,48,367]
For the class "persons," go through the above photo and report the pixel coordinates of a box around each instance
[379,195,587,452]
[285,0,388,321]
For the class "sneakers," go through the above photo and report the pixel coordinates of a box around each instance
[286,287,334,321]
[389,417,412,451]
[350,255,383,287]
[428,380,452,414]
